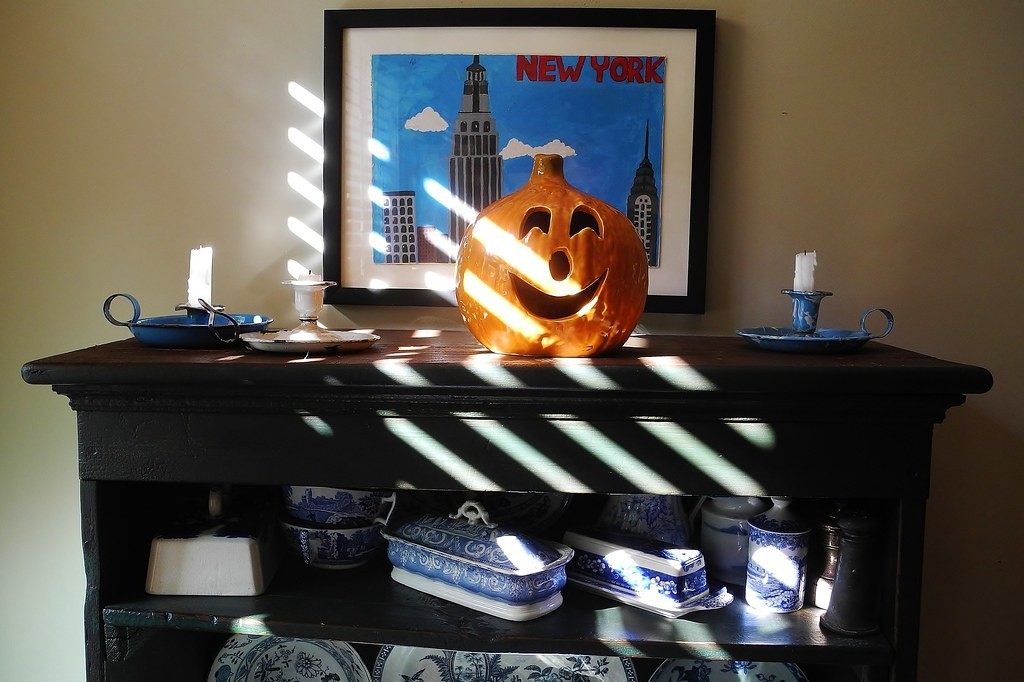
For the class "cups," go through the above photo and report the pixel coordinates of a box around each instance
[744,525,809,614]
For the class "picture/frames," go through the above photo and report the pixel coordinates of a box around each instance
[322,9,715,316]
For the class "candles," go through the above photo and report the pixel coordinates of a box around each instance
[793,249,818,292]
[187,244,213,308]
[295,269,324,317]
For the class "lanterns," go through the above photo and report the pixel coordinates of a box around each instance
[455,154,650,357]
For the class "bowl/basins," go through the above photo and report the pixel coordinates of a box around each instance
[702,512,758,588]
[283,483,396,528]
[282,521,387,570]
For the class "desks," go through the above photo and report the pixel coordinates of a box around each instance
[20,329,993,682]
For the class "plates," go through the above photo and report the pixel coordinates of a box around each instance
[206,631,806,682]
